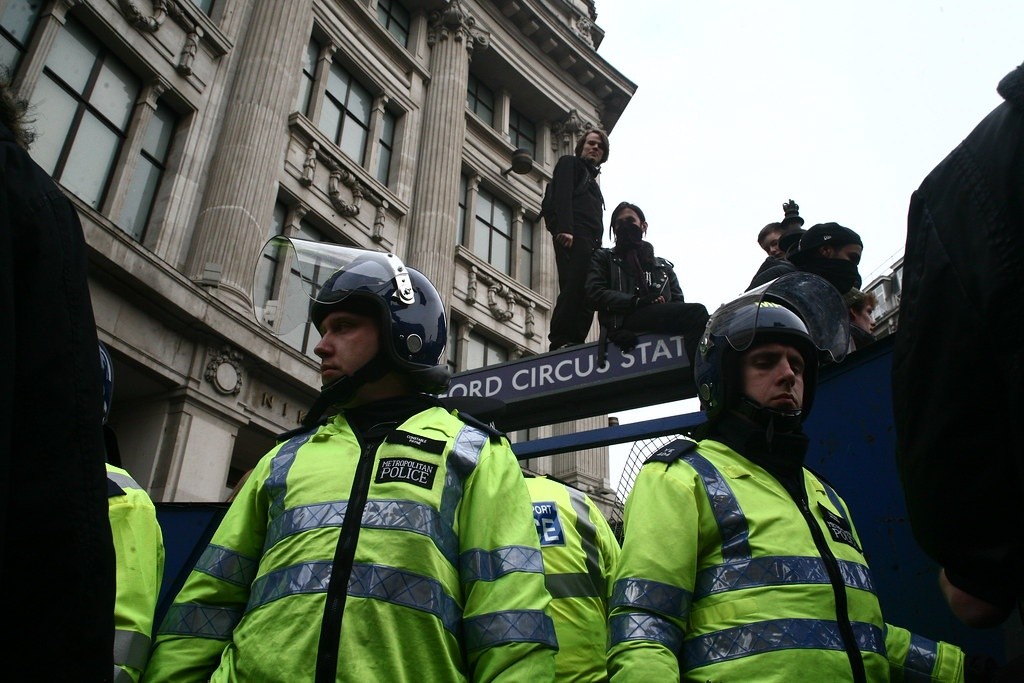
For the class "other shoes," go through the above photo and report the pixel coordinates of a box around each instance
[549,342,584,352]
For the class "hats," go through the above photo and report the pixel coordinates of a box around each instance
[785,221,863,260]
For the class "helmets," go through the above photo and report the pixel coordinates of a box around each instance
[310,253,453,395]
[697,300,824,438]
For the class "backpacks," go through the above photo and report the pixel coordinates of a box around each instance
[536,155,591,236]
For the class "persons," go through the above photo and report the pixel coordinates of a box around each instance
[891,64,1024,683]
[542,130,609,352]
[0,62,115,683]
[746,222,879,354]
[98,337,166,683]
[608,295,966,683]
[585,202,709,398]
[523,476,620,683]
[141,235,559,683]
